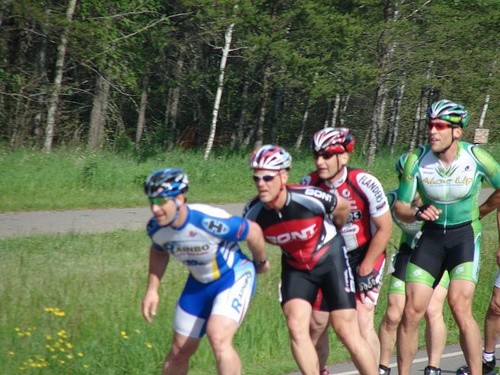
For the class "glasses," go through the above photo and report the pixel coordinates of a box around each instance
[314,152,336,159]
[252,173,282,182]
[427,122,452,130]
[150,197,169,205]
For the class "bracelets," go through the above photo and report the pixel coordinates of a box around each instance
[252,259,267,269]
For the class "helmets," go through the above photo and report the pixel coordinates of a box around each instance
[397,153,407,174]
[312,127,354,153]
[248,144,291,170]
[427,99,469,127]
[145,168,189,199]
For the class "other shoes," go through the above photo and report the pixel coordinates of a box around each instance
[484,355,496,375]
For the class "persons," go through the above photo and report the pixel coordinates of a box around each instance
[457,207,500,374]
[242,145,379,375]
[143,168,269,375]
[298,127,392,375]
[394,100,500,374]
[376,152,450,375]
